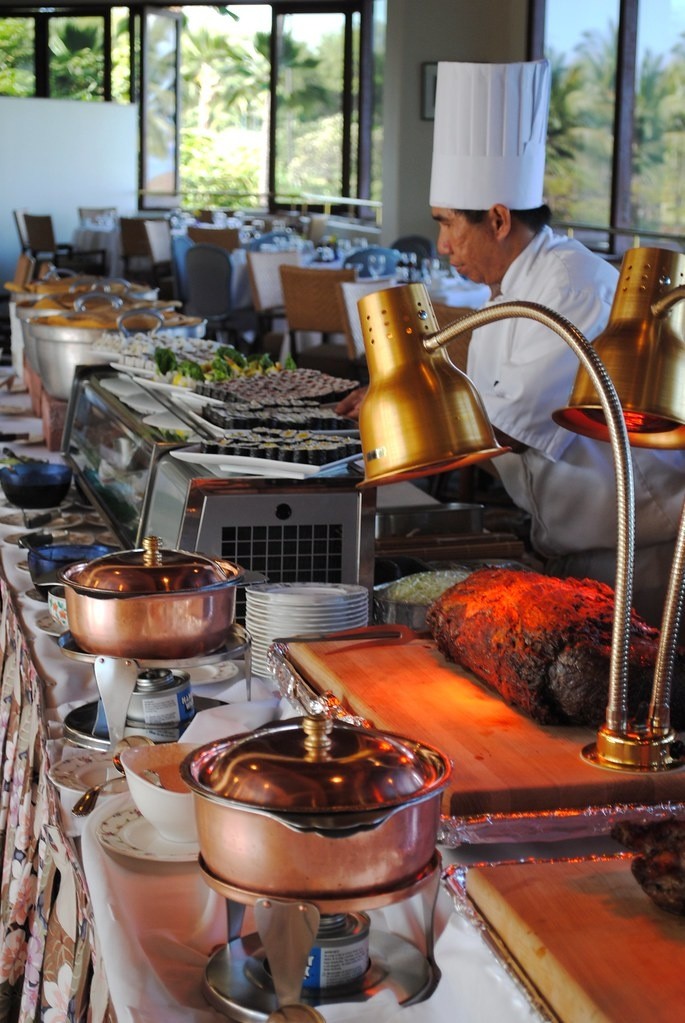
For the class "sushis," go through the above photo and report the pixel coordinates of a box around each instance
[119,353,219,375]
[193,368,361,466]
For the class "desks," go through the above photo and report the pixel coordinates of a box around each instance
[279,275,492,364]
[0,366,545,1023]
[70,225,124,278]
[209,239,317,344]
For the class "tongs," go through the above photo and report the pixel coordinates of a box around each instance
[20,501,73,529]
[18,529,70,546]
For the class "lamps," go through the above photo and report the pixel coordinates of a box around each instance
[550,248,685,774]
[355,282,685,774]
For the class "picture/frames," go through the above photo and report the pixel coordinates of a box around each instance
[419,60,437,121]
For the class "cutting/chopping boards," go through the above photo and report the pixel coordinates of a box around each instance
[464,857,685,1023]
[286,640,685,814]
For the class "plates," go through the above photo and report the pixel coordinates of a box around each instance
[0,531,95,550]
[0,511,86,530]
[142,660,239,686]
[109,362,362,480]
[0,499,73,512]
[15,560,29,572]
[243,581,369,679]
[48,750,129,796]
[97,807,201,862]
[33,613,67,637]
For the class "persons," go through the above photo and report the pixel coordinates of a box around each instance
[336,57,685,653]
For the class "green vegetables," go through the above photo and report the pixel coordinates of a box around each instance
[154,346,297,384]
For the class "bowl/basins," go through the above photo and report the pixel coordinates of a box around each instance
[0,463,73,509]
[121,743,202,842]
[27,544,121,591]
[48,586,67,626]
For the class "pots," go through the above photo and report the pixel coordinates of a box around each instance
[58,535,245,660]
[13,269,206,401]
[177,687,454,898]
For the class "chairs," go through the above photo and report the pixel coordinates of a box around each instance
[342,244,397,278]
[432,302,475,373]
[78,206,119,225]
[246,249,303,364]
[183,246,242,351]
[334,279,396,359]
[278,262,369,387]
[120,216,167,286]
[22,214,110,280]
[186,226,240,253]
[143,219,174,300]
[13,209,31,254]
[392,235,434,270]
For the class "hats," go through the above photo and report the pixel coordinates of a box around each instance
[428,56,551,211]
